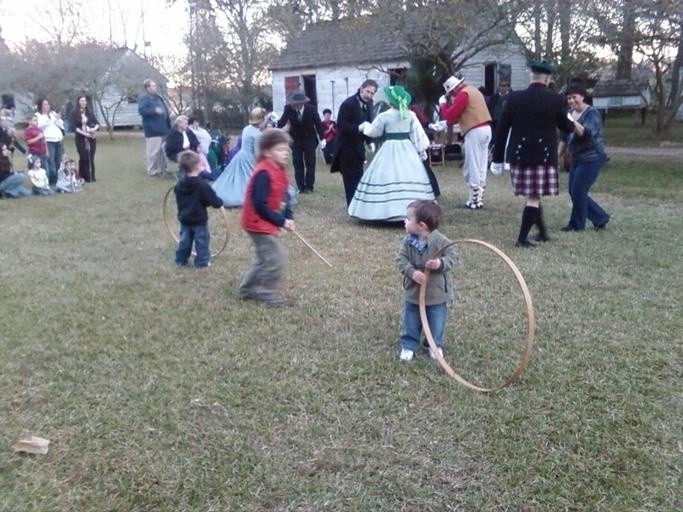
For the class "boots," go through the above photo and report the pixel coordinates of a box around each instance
[466,185,486,209]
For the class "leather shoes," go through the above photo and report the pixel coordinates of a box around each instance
[297,186,313,195]
[514,216,610,246]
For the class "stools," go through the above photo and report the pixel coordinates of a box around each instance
[428,143,445,168]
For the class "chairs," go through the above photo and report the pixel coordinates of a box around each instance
[161,141,180,181]
[314,125,328,166]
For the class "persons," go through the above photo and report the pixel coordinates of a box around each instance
[411,71,513,210]
[489,61,577,249]
[346,83,440,224]
[209,106,268,207]
[174,151,223,267]
[238,129,297,309]
[557,84,610,232]
[395,198,458,363]
[137,79,171,176]
[321,108,338,164]
[164,109,278,180]
[330,78,378,207]
[272,92,325,194]
[0,95,100,199]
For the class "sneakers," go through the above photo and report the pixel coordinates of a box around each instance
[148,166,174,179]
[430,346,443,360]
[399,347,414,361]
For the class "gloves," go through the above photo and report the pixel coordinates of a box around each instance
[490,162,503,174]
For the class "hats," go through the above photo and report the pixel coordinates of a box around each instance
[441,76,466,98]
[384,85,413,110]
[530,60,555,74]
[247,107,266,125]
[286,90,311,108]
